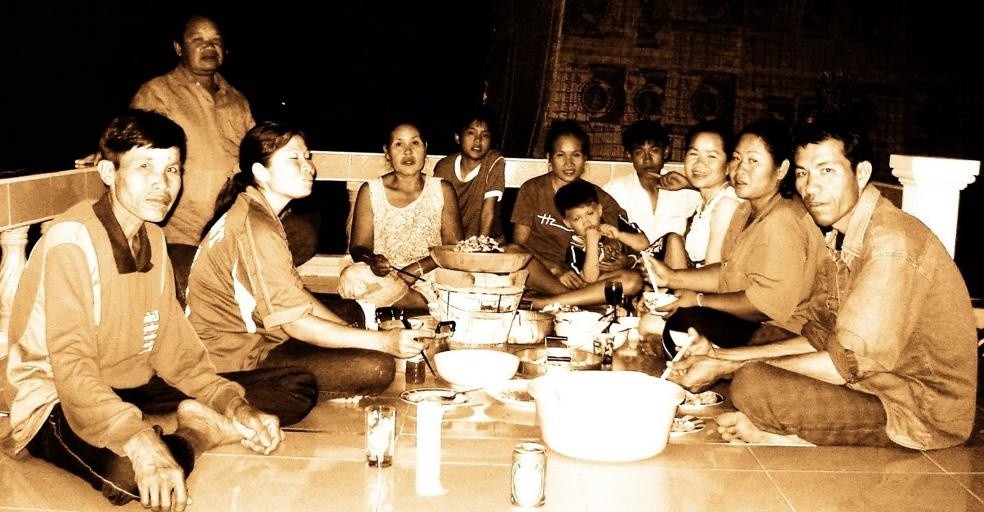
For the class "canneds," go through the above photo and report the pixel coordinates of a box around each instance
[509,442,548,508]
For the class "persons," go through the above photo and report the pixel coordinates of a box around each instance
[602,117,704,246]
[74,13,318,316]
[637,118,746,339]
[714,116,979,451]
[554,179,688,284]
[183,120,438,396]
[1,105,320,511]
[432,109,507,248]
[638,118,828,360]
[335,114,477,311]
[509,117,645,310]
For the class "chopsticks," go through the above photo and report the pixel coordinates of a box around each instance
[661,336,694,377]
[640,249,658,293]
[400,317,436,377]
[361,254,426,281]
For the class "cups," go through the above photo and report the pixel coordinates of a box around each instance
[365,405,393,466]
[417,460,440,495]
[416,401,440,460]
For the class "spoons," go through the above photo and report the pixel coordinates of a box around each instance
[441,389,486,399]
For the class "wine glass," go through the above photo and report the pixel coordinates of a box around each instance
[604,281,624,324]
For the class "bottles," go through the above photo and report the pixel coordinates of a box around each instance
[593,336,601,355]
[407,326,426,384]
[604,337,614,368]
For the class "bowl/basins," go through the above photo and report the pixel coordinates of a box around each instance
[429,245,531,273]
[644,294,675,316]
[433,349,520,389]
[669,330,722,349]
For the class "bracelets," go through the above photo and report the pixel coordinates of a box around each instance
[416,260,425,276]
[696,292,704,307]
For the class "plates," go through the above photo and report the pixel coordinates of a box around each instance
[617,317,670,330]
[488,380,535,410]
[682,389,723,408]
[400,387,465,404]
[373,277,409,306]
[554,311,609,333]
[669,413,706,434]
[562,322,630,350]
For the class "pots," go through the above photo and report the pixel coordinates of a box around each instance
[508,300,557,345]
[376,306,456,352]
[512,337,603,376]
[530,371,685,461]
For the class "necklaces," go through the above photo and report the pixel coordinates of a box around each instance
[697,179,732,220]
